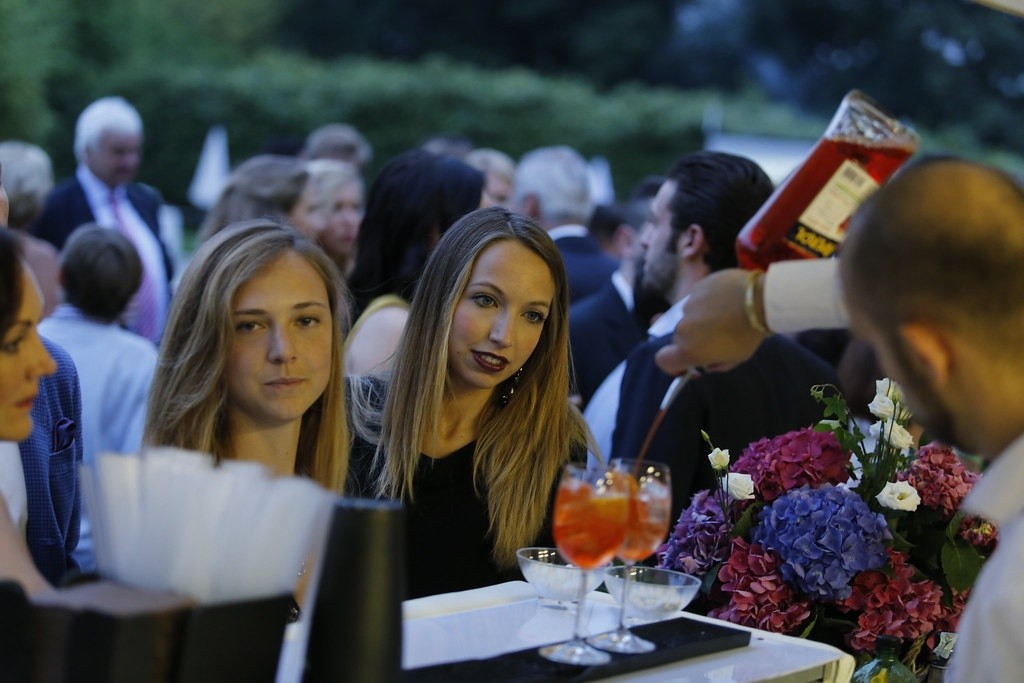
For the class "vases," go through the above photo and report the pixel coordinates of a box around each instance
[850,636,922,683]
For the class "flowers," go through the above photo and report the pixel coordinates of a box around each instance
[653,374,999,683]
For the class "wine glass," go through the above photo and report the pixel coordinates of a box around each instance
[540,462,641,665]
[584,459,672,653]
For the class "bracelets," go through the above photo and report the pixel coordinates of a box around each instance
[745,270,770,337]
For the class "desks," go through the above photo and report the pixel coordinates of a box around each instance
[277,581,856,683]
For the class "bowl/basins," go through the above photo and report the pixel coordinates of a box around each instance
[601,564,701,621]
[517,547,610,609]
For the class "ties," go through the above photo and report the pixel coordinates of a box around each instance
[104,189,157,341]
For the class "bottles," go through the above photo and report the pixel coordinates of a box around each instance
[846,635,918,682]
[679,89,922,383]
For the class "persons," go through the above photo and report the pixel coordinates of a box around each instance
[656,156,1024,683]
[0,97,884,621]
